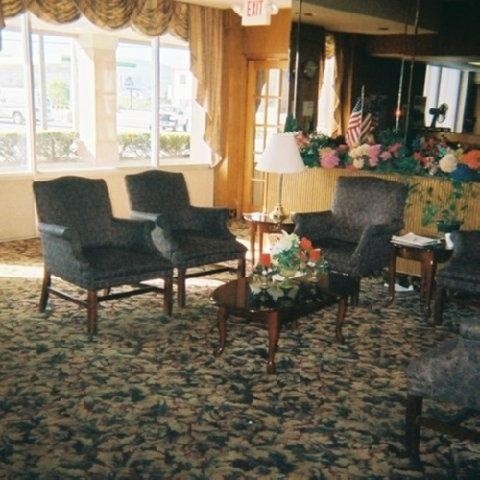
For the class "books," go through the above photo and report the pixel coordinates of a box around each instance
[391,231,442,249]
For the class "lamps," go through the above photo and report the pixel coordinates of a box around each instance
[255,134,305,219]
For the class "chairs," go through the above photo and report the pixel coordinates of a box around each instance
[30,175,174,336]
[405,319,480,457]
[432,230,480,325]
[294,176,408,306]
[124,171,248,307]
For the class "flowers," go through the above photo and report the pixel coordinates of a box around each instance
[284,114,480,222]
[250,233,328,308]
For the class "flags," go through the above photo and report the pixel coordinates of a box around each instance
[345,95,377,147]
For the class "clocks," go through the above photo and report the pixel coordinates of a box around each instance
[303,59,316,79]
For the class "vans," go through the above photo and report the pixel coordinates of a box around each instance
[0,87,55,126]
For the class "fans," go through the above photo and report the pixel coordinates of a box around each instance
[430,104,448,128]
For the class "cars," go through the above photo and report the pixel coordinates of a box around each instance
[158,104,190,134]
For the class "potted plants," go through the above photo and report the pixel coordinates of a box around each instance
[420,176,480,233]
